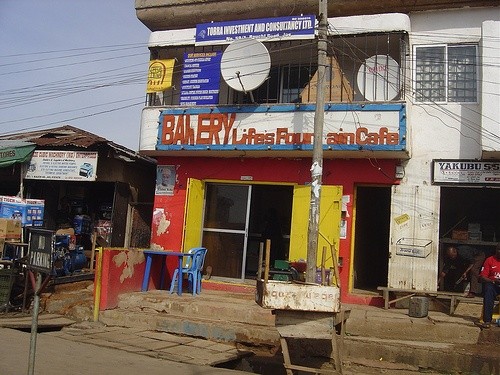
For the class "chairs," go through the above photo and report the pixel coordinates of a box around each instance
[169,247,208,296]
[0,260,14,317]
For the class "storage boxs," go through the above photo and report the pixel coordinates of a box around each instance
[0,218,22,259]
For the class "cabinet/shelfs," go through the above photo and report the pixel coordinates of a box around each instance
[441,237,500,295]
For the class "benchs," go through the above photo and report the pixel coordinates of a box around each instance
[377,286,463,316]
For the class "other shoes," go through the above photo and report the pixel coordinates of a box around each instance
[484,322,490,328]
[466,293,474,297]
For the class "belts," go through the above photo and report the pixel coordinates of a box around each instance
[476,249,483,257]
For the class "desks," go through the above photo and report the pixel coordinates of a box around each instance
[142,251,198,297]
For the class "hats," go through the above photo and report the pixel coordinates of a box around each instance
[161,169,171,176]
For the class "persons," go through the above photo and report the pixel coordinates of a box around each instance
[157,168,174,191]
[440,246,487,298]
[478,244,500,328]
